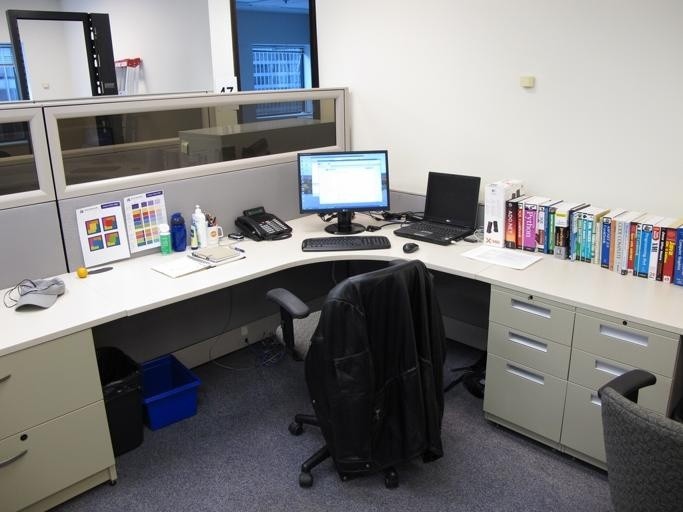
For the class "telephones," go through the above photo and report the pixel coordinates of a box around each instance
[235,207,292,241]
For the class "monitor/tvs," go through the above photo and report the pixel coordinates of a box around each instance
[297,150,390,235]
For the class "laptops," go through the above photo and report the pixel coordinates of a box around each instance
[393,172,480,245]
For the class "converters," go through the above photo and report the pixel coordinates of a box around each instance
[410,215,423,220]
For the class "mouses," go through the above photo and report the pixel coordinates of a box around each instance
[403,243,418,253]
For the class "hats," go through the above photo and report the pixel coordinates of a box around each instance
[14,278,65,310]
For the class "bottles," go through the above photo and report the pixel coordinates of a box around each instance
[192,205,207,248]
[159,212,185,255]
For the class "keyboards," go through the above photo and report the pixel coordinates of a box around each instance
[301,236,390,253]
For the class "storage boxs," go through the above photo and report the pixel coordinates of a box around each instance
[136,353,201,431]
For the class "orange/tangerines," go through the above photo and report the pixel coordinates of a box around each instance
[78,268,87,277]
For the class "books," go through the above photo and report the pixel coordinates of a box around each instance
[187,249,246,269]
[150,257,208,278]
[191,244,239,262]
[483,176,682,288]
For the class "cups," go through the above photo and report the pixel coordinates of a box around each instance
[208,224,223,244]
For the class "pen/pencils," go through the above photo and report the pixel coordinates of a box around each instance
[235,247,244,253]
[204,211,217,228]
[88,267,114,274]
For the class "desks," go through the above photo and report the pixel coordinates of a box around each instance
[0,211,682,512]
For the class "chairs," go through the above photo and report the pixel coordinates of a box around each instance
[597,370,682,512]
[265,259,446,488]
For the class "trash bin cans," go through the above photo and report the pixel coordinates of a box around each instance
[95,345,144,458]
